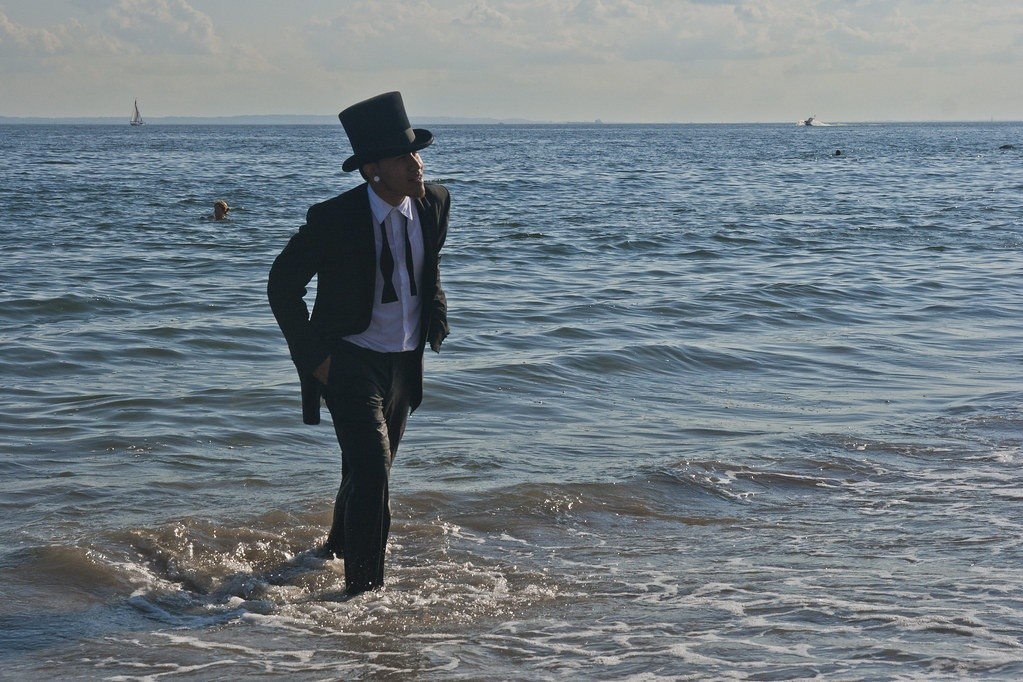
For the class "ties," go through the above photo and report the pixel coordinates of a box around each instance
[379,216,418,304]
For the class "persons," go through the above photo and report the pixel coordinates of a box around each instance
[267,92,452,598]
[214,200,228,220]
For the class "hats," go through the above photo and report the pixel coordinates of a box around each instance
[338,91,433,172]
